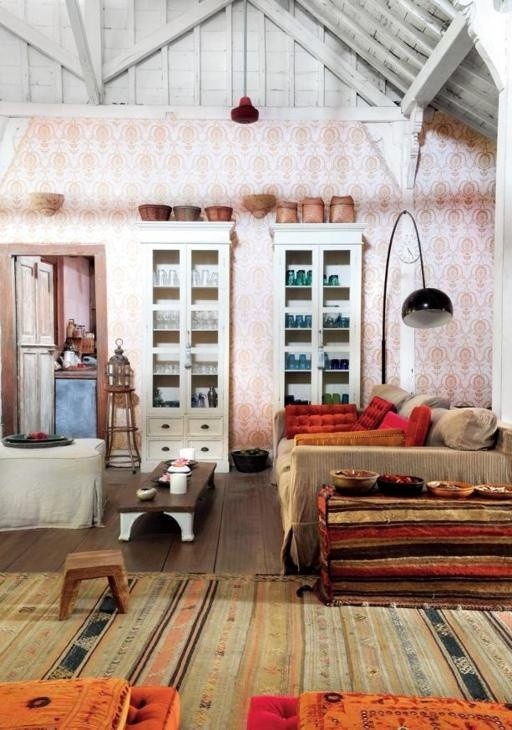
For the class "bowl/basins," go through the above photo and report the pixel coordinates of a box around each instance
[330,470,474,499]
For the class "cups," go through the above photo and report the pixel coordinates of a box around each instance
[150,269,218,373]
[169,474,188,494]
[180,448,194,461]
[285,269,349,405]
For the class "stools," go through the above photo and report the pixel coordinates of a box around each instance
[59,549,129,619]
[107,388,141,473]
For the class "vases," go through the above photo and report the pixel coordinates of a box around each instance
[231,450,268,470]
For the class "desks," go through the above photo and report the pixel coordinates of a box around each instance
[318,485,512,605]
[0,439,105,531]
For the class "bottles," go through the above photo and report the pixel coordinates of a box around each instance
[58,351,75,367]
[66,318,95,353]
[154,389,162,406]
[191,386,217,408]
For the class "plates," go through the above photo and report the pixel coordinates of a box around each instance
[6,434,65,441]
[1,438,73,448]
[474,482,511,497]
[151,458,197,486]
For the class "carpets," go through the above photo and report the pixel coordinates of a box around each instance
[0,573,511,730]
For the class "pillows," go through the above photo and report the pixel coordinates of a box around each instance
[425,407,497,451]
[349,396,396,430]
[400,396,450,419]
[378,411,411,430]
[293,428,406,446]
[285,404,356,439]
[407,406,438,445]
[367,385,410,409]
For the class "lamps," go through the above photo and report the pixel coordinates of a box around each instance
[231,2,258,123]
[382,210,453,383]
[136,223,237,474]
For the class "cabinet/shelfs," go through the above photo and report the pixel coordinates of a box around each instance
[53,369,97,439]
[270,235,365,406]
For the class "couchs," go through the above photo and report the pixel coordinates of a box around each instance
[271,385,512,578]
